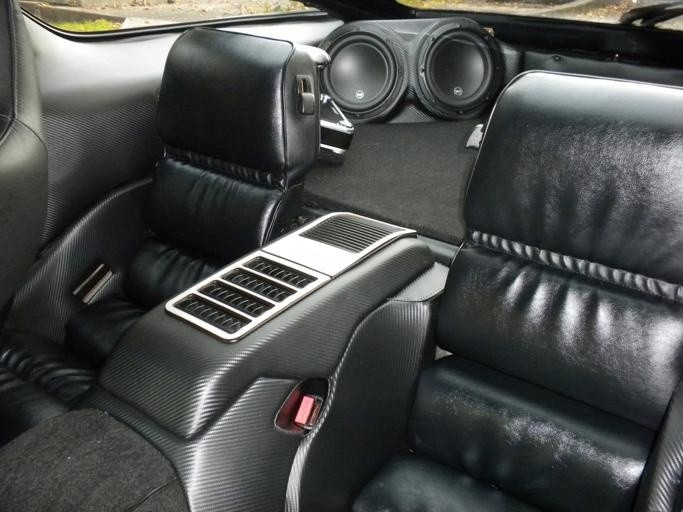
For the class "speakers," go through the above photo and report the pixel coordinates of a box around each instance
[318,18,503,125]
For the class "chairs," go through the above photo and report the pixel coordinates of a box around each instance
[286,68,682,512]
[0,26,320,447]
